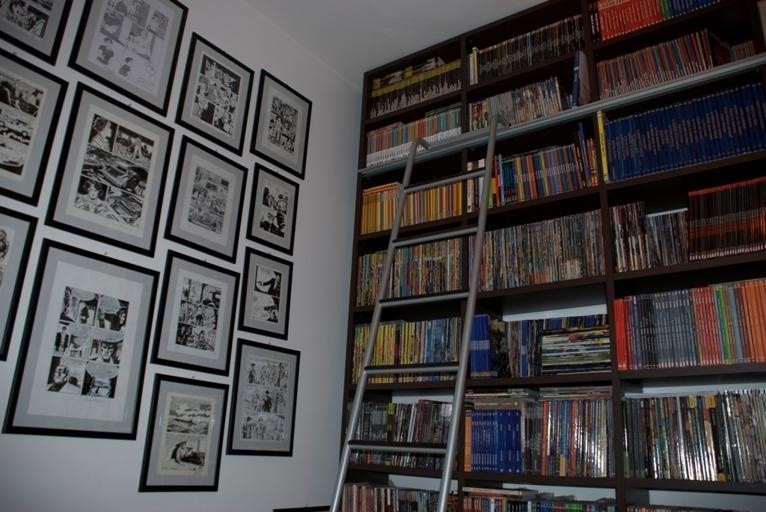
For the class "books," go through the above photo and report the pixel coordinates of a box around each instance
[341,11,615,511]
[596,0,766,511]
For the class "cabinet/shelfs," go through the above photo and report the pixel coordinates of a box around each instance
[334,0,766,512]
